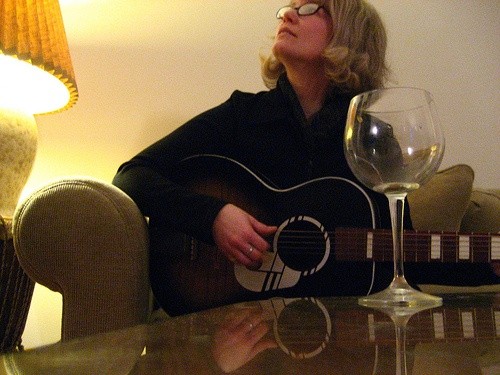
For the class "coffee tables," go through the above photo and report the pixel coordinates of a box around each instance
[0,296,500,375]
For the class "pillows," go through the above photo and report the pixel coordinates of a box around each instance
[405,163,476,230]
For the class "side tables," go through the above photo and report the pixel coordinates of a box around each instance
[0,219,35,352]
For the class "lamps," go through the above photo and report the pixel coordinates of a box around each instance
[0,0,79,237]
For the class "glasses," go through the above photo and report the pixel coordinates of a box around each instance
[276,2,325,19]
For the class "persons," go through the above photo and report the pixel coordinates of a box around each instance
[120,0,500,315]
[127,296,416,375]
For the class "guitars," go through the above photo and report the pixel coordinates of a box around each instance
[149,151,500,314]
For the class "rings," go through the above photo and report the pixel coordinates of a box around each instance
[245,246,255,257]
[242,320,255,330]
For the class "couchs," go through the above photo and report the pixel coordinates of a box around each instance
[11,176,500,344]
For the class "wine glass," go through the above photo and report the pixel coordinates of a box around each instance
[345,86,447,309]
